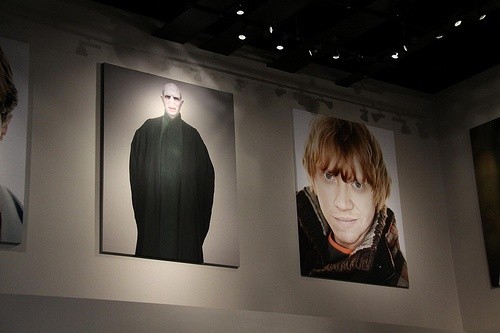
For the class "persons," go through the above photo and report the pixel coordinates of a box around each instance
[127,80,218,265]
[0,48,26,244]
[293,110,410,289]
